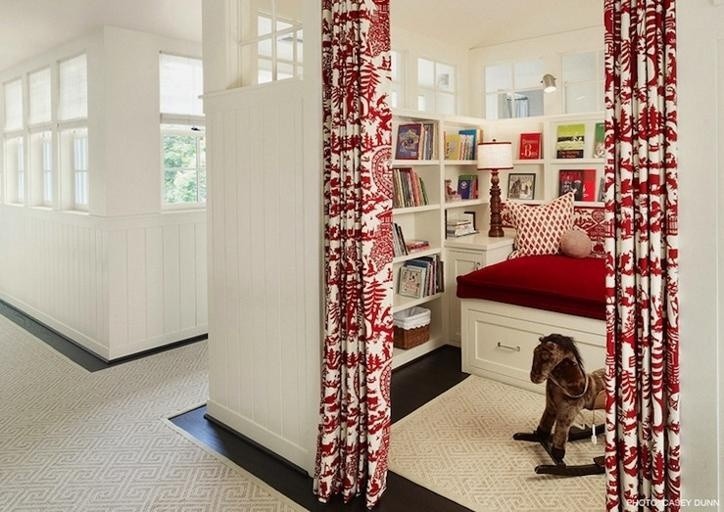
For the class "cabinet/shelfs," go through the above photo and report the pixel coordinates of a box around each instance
[489,112,607,230]
[392,109,490,370]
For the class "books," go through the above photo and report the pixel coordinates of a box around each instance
[389,123,604,301]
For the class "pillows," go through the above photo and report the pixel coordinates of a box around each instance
[509,192,577,256]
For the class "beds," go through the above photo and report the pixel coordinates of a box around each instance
[456,204,607,395]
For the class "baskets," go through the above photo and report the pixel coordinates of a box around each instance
[392,306,432,350]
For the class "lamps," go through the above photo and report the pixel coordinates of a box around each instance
[476,139,514,237]
[540,73,558,93]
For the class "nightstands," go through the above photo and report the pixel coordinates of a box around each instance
[444,234,513,348]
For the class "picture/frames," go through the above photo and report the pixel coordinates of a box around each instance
[506,172,536,200]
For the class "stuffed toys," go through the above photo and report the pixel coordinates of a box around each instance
[530,333,606,470]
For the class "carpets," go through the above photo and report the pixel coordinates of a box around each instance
[388,373,607,512]
[0,319,308,512]
